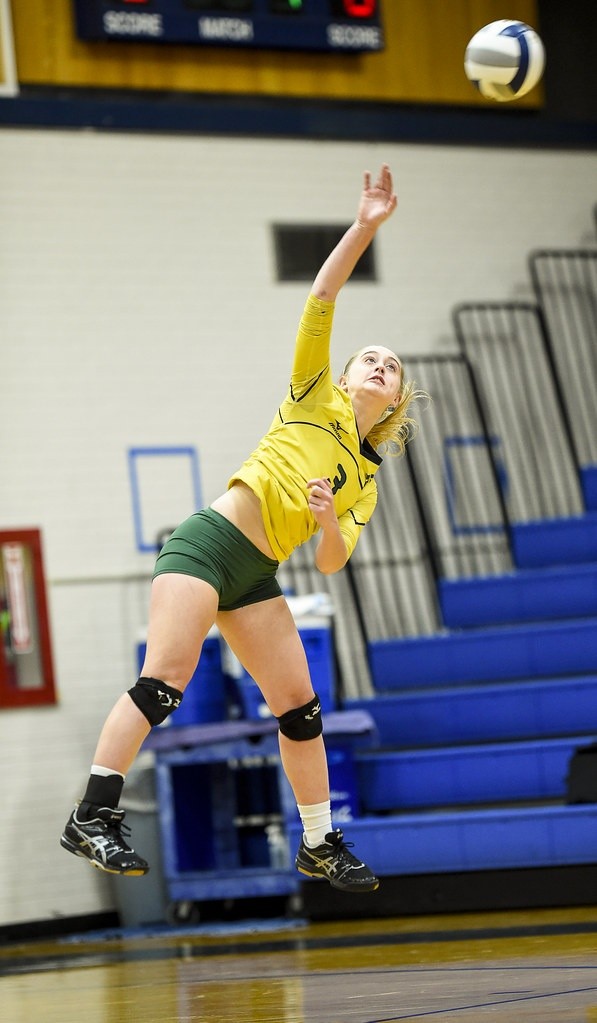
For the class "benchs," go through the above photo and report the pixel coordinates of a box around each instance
[296,466,597,914]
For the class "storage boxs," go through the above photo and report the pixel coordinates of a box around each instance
[135,588,335,732]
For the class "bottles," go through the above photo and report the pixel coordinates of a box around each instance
[266,824,284,870]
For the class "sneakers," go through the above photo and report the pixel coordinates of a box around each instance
[295,828,380,893]
[60,799,150,876]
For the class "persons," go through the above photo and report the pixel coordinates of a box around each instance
[59,165,431,893]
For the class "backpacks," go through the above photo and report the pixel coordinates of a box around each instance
[563,743,597,805]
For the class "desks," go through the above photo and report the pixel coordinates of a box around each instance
[129,711,377,922]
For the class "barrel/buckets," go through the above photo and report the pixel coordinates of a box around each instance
[234,591,337,720]
[134,622,228,732]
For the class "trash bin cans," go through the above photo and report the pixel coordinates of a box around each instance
[228,627,335,716]
[137,635,231,724]
[106,767,170,929]
